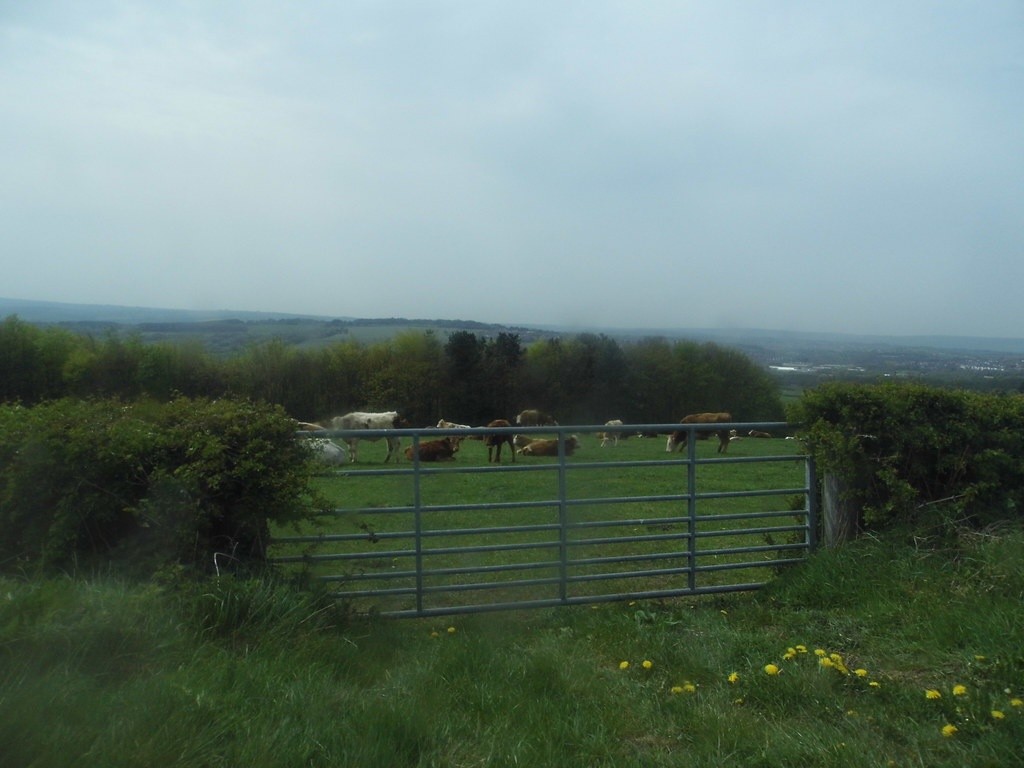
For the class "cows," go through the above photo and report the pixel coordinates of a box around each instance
[318,409,794,464]
